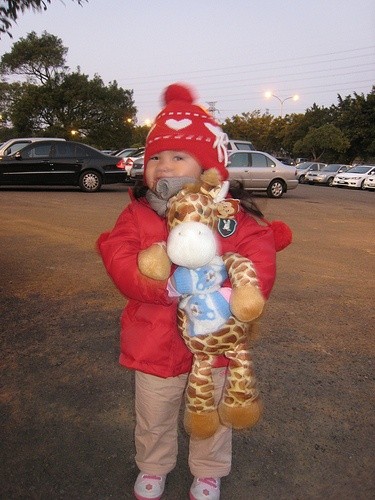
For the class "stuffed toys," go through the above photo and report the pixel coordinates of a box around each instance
[138,163,265,441]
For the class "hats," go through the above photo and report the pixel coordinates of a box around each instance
[143,84,229,181]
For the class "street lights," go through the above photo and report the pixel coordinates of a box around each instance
[266,91,300,116]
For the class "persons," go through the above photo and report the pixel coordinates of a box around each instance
[97,83,292,500]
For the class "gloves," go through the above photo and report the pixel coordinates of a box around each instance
[174,256,228,295]
[179,287,233,337]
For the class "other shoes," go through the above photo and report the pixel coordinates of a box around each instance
[134,471,166,500]
[189,475,221,500]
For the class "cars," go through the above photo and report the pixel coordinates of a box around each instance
[332,164,375,192]
[294,161,328,184]
[129,148,299,199]
[0,140,128,193]
[101,139,299,178]
[304,163,357,187]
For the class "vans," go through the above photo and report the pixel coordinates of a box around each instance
[0,138,72,160]
